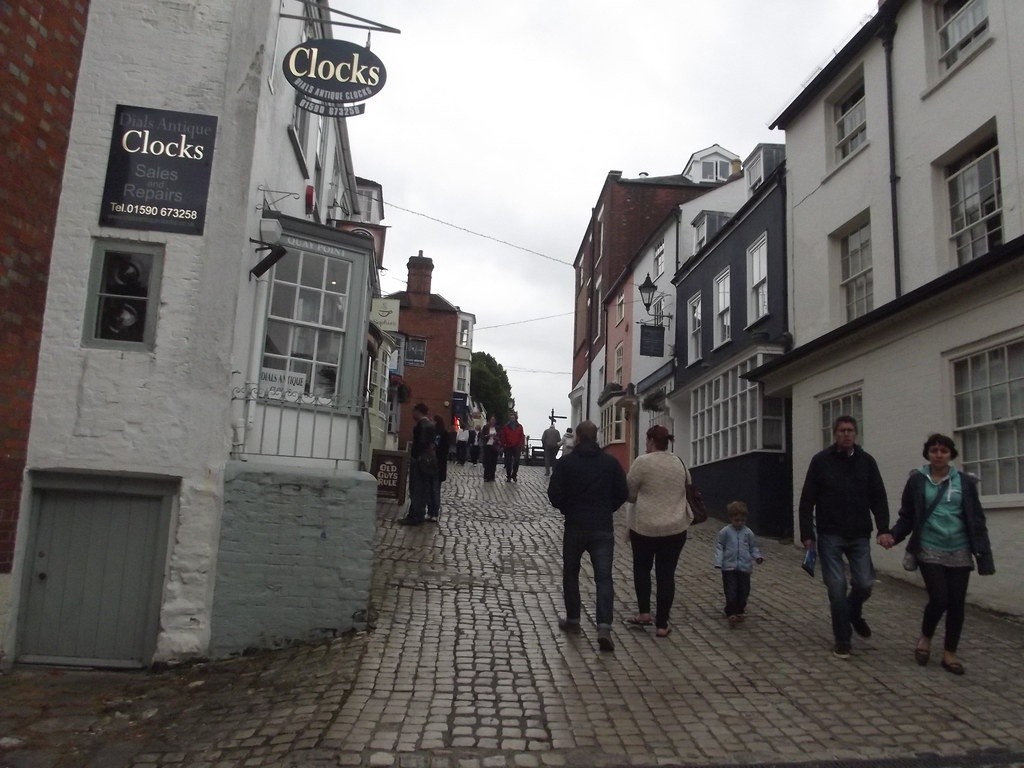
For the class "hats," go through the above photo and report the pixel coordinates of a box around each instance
[415,402,428,416]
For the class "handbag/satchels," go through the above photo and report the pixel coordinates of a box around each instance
[419,447,438,473]
[678,456,708,525]
[902,527,920,571]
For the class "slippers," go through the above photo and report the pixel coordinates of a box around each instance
[656,628,671,637]
[627,617,653,626]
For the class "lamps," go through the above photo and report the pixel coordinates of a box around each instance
[250,236,287,278]
[638,272,672,318]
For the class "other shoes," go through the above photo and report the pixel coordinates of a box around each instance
[832,643,850,659]
[427,515,437,522]
[507,477,511,482]
[398,515,424,526]
[737,616,744,622]
[914,639,931,665]
[851,618,871,638]
[597,628,615,651]
[513,477,517,481]
[729,615,737,626]
[474,463,477,466]
[559,619,581,633]
[941,659,964,675]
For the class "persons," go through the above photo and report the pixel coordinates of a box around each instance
[501,414,524,483]
[878,432,996,676]
[541,424,576,476]
[799,414,892,655]
[397,402,450,525]
[627,424,694,637]
[454,423,482,467]
[481,414,501,482]
[546,419,628,651]
[714,502,764,626]
